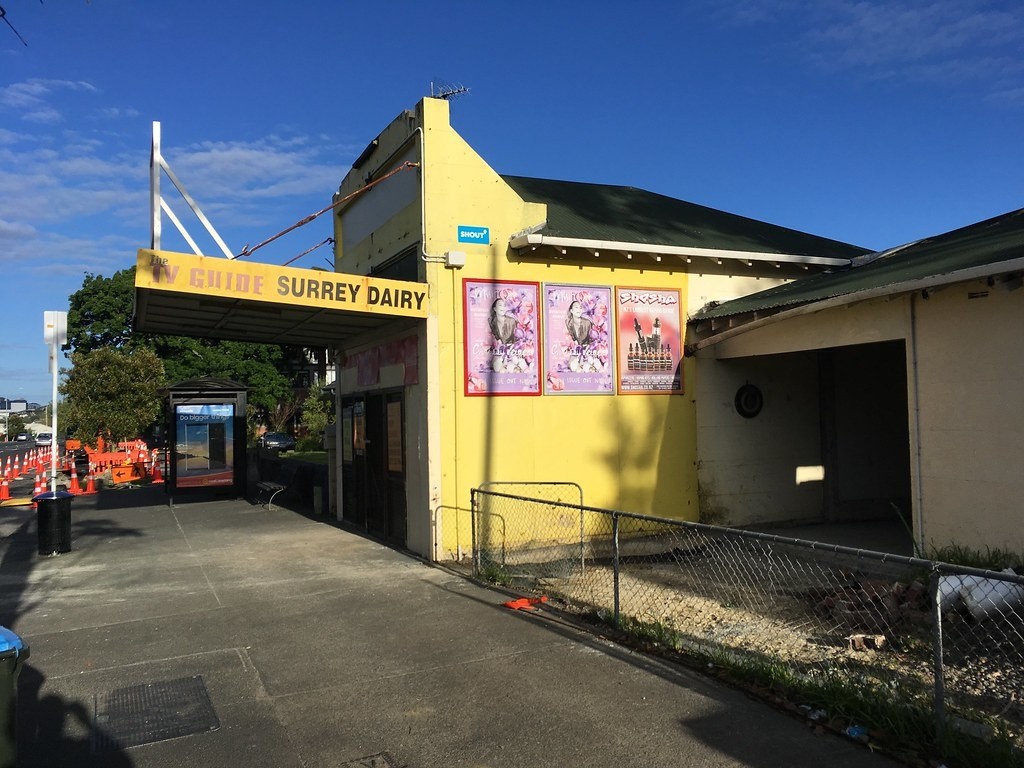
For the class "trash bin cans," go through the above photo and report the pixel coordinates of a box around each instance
[0,625,30,768]
[30,491,75,556]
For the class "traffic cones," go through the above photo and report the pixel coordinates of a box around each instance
[65,459,84,496]
[39,470,48,492]
[28,469,42,508]
[0,467,14,501]
[0,438,165,484]
[83,464,100,495]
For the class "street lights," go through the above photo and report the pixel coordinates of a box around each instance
[3,395,10,442]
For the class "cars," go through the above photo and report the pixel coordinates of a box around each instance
[257,432,297,453]
[16,432,31,442]
[35,432,53,447]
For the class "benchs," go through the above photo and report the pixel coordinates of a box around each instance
[250,462,302,514]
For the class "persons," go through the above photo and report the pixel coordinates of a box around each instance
[566,301,592,346]
[488,298,517,345]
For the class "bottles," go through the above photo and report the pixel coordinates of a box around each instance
[628,316,675,373]
